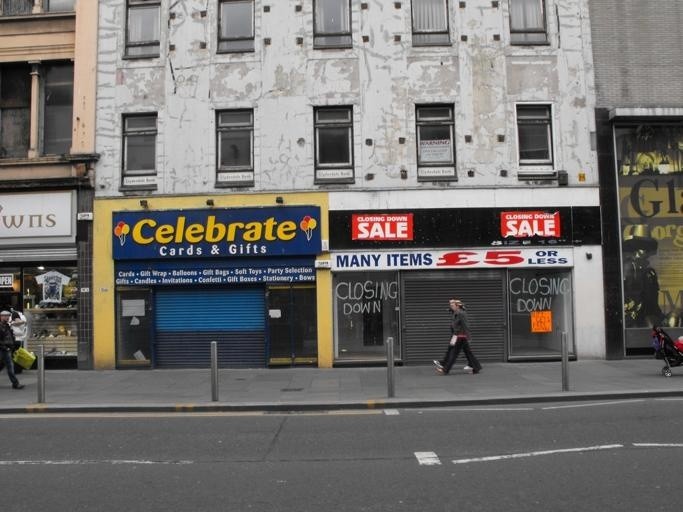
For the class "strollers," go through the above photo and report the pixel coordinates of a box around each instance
[649,325,683,378]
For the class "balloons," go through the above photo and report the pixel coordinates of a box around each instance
[299,214,317,233]
[113,222,129,237]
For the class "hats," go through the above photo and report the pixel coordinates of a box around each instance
[454,300,464,306]
[0,311,11,316]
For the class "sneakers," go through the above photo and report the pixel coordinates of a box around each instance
[463,365,481,374]
[432,359,447,375]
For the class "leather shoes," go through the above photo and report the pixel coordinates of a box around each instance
[13,381,25,389]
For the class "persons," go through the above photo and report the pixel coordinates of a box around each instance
[0,311,26,389]
[8,306,27,335]
[432,298,474,370]
[435,299,483,375]
[9,311,24,375]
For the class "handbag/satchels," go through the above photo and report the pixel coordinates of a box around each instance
[449,335,458,346]
[13,347,36,370]
